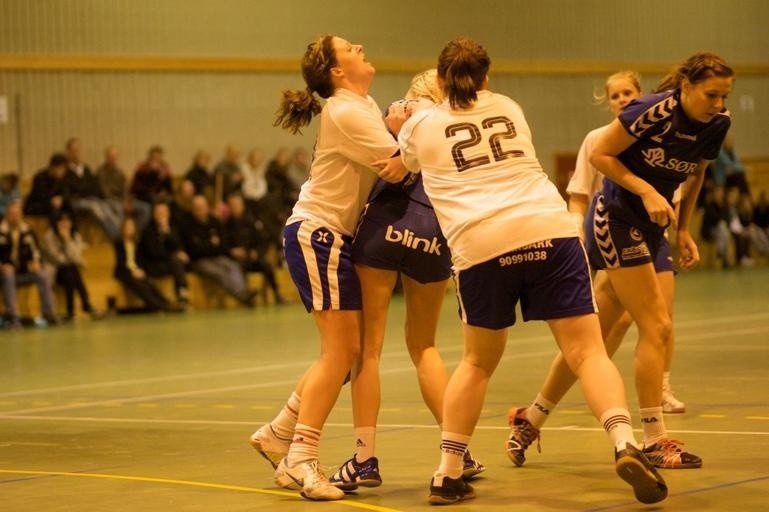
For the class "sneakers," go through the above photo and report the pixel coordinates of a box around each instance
[463,450,486,478]
[505,407,541,467]
[660,389,686,413]
[250,423,294,470]
[641,437,702,469]
[328,454,382,491]
[427,471,477,503]
[614,438,668,504]
[273,456,346,501]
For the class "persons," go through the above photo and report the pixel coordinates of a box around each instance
[692,135,768,273]
[248,33,413,499]
[504,53,737,470]
[396,36,668,505]
[328,68,486,489]
[565,72,686,411]
[2,131,312,322]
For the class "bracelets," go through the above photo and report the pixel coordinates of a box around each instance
[404,99,420,114]
